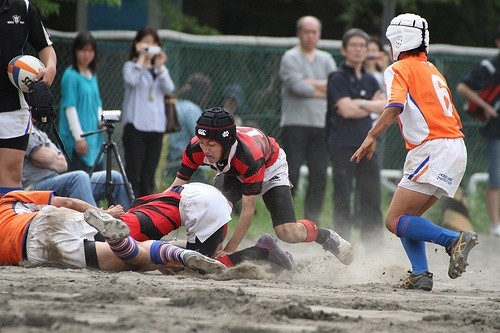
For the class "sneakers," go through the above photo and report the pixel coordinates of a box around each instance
[84,209,130,240]
[445,232,479,279]
[490,226,500,238]
[181,250,228,275]
[397,270,433,291]
[255,233,293,270]
[319,228,354,266]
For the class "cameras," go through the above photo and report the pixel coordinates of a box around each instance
[143,45,162,55]
[101,110,121,121]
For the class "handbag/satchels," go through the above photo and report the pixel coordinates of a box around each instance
[164,93,180,133]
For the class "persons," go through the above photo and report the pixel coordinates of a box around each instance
[326,27,389,252]
[455,37,500,237]
[162,72,355,266]
[117,181,293,271]
[0,0,58,190]
[120,27,175,201]
[0,190,226,275]
[163,72,213,185]
[278,16,337,227]
[21,120,133,214]
[221,82,247,126]
[58,32,105,176]
[349,14,479,292]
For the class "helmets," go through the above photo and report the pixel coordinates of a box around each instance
[195,107,236,161]
[385,13,429,63]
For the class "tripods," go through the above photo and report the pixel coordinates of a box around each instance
[80,122,135,207]
